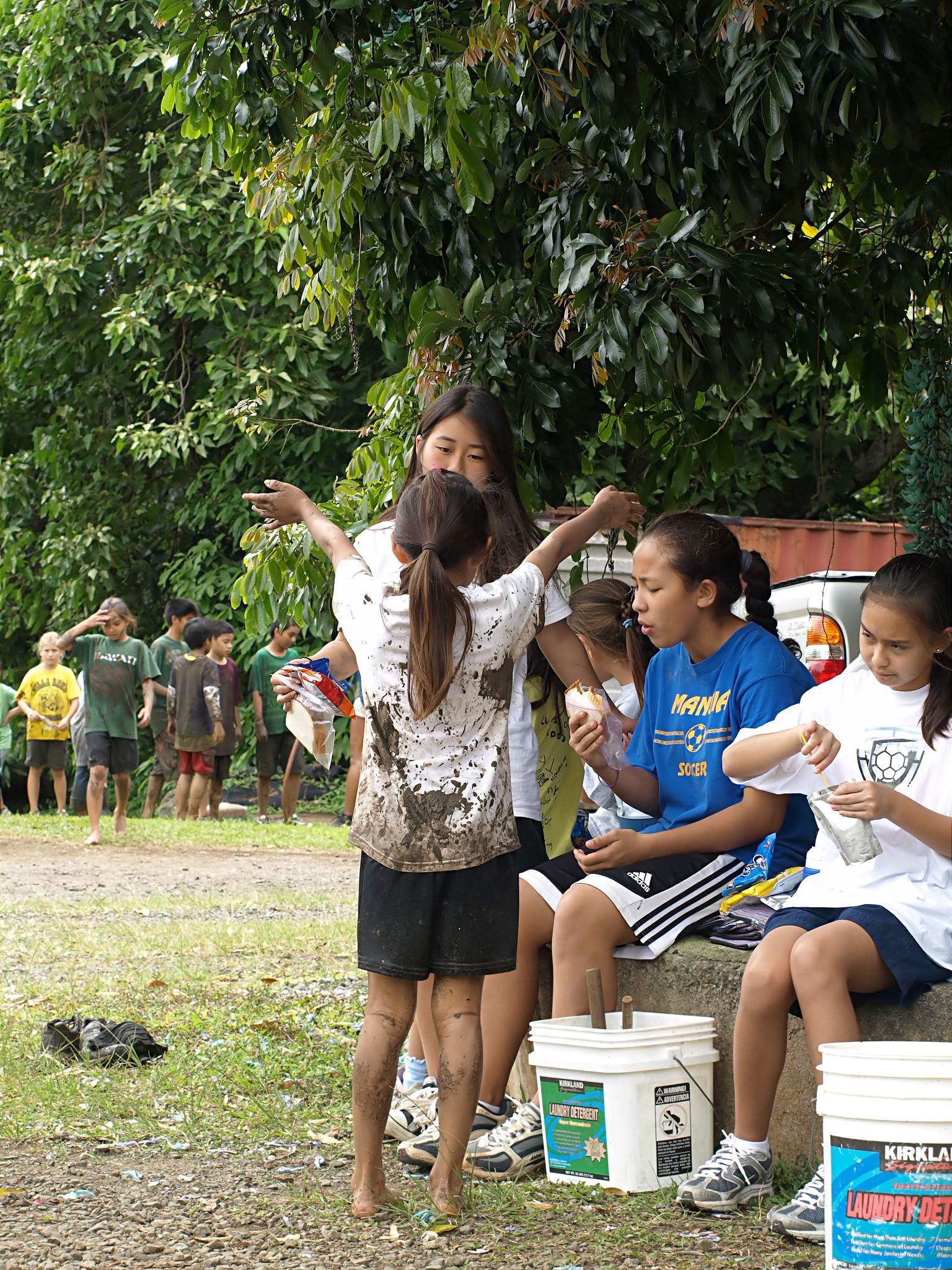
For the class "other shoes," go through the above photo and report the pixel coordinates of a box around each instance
[281,814,311,827]
[257,815,270,825]
[393,1066,425,1099]
[331,814,351,828]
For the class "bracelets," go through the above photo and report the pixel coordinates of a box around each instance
[598,762,620,789]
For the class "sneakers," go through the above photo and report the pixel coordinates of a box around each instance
[675,1129,774,1211]
[396,1098,515,1166]
[462,1094,544,1181]
[770,1163,825,1240]
[385,1077,439,1141]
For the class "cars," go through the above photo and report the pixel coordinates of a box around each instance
[732,571,882,688]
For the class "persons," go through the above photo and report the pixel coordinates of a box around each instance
[243,467,646,1224]
[675,553,952,1243]
[56,596,162,845]
[398,508,819,1179]
[269,385,639,1111]
[0,596,313,828]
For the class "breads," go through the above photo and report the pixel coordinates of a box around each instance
[565,679,611,728]
[285,693,327,754]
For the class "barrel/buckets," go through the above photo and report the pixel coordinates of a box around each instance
[528,1011,720,1195]
[808,1041,951,1270]
